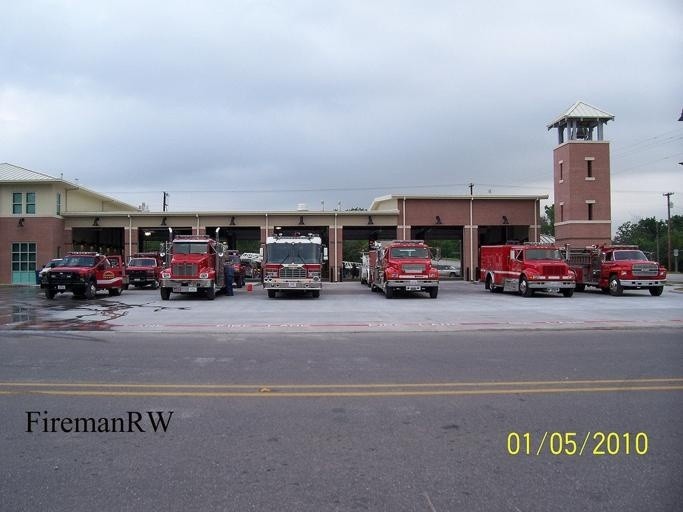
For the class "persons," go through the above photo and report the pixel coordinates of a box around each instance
[222,258,235,296]
[238,259,245,286]
[229,258,242,289]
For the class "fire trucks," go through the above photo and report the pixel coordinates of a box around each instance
[34,225,669,302]
